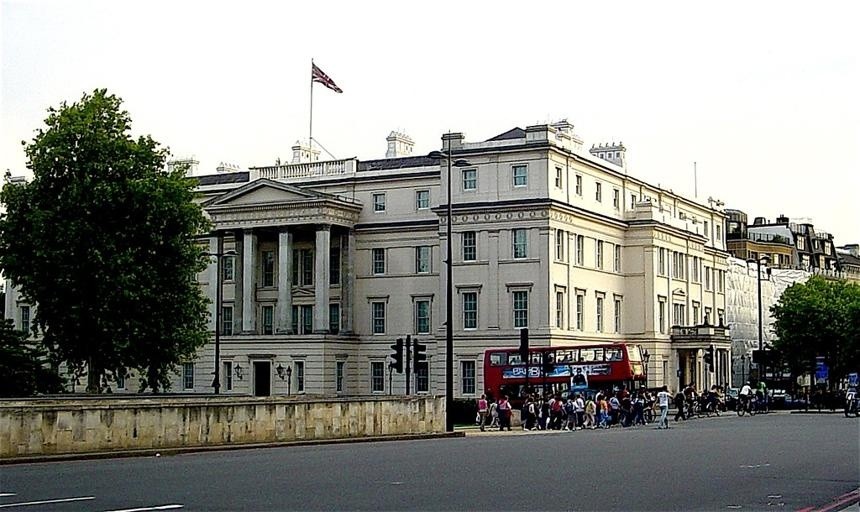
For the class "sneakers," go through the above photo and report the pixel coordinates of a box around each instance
[659,424,670,429]
[676,410,722,422]
[523,422,645,431]
[479,423,512,431]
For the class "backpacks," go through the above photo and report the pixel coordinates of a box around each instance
[566,401,574,413]
[554,400,560,412]
[522,404,531,418]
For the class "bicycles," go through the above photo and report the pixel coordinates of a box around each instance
[632,390,777,418]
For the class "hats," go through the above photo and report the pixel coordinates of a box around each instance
[746,381,750,384]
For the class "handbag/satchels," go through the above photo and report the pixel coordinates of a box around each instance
[479,409,492,417]
[633,402,640,411]
[563,414,568,420]
[602,412,608,418]
[505,408,511,418]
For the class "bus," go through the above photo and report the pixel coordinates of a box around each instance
[484,342,648,399]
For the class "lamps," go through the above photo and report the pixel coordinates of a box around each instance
[234,363,242,380]
[276,363,284,379]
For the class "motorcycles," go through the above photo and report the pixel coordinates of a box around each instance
[843,375,860,418]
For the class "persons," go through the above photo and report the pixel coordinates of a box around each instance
[673,381,770,421]
[796,372,857,411]
[478,381,674,429]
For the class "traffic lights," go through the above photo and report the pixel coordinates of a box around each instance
[391,335,427,378]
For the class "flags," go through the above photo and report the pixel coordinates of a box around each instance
[313,64,344,94]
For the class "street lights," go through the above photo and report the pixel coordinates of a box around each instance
[428,127,470,431]
[746,251,772,394]
